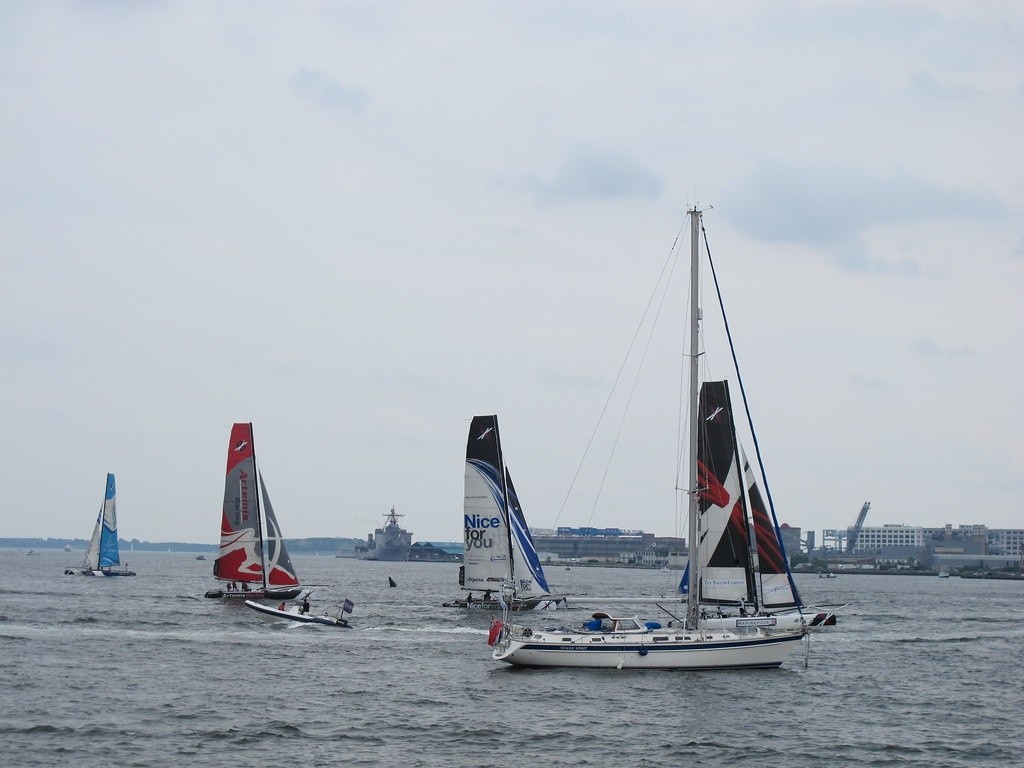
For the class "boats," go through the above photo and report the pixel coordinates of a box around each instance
[939,572,949,577]
[245,590,355,630]
[818,572,837,578]
[196,555,206,560]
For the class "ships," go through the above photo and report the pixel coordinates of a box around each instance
[353,505,464,562]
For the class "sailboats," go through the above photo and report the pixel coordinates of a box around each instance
[203,423,314,598]
[487,200,852,668]
[65,473,136,577]
[443,414,563,610]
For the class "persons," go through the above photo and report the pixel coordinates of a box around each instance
[716,606,722,615]
[278,602,285,611]
[739,597,745,617]
[301,599,309,615]
[226,582,231,591]
[242,582,251,592]
[467,593,472,601]
[232,580,239,592]
[484,589,491,601]
[700,607,711,619]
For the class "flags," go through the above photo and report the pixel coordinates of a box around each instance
[488,614,503,647]
